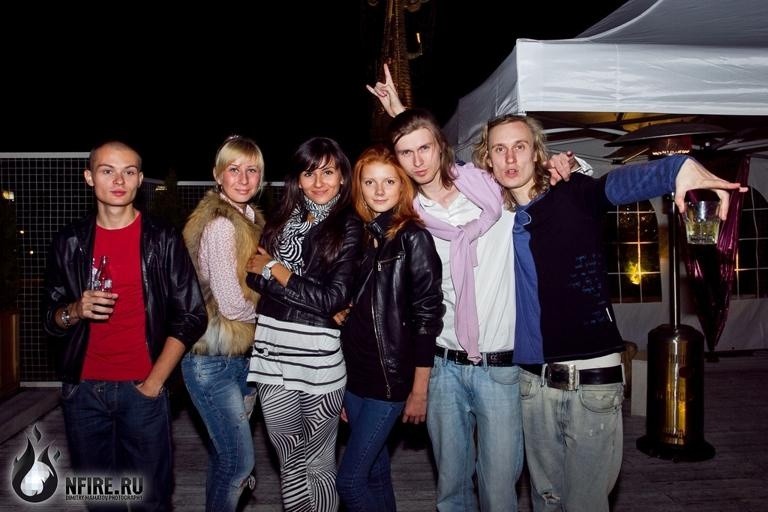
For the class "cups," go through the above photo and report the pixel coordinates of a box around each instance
[682,201,720,245]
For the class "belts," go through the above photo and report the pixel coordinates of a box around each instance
[517,364,622,391]
[435,345,514,366]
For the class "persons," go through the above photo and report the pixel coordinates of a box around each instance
[181,136,264,512]
[365,62,750,512]
[244,134,364,512]
[388,105,595,512]
[39,138,208,512]
[334,145,447,512]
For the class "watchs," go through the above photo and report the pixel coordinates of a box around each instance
[60,303,72,330]
[261,260,279,281]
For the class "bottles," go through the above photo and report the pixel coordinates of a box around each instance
[91,256,112,322]
